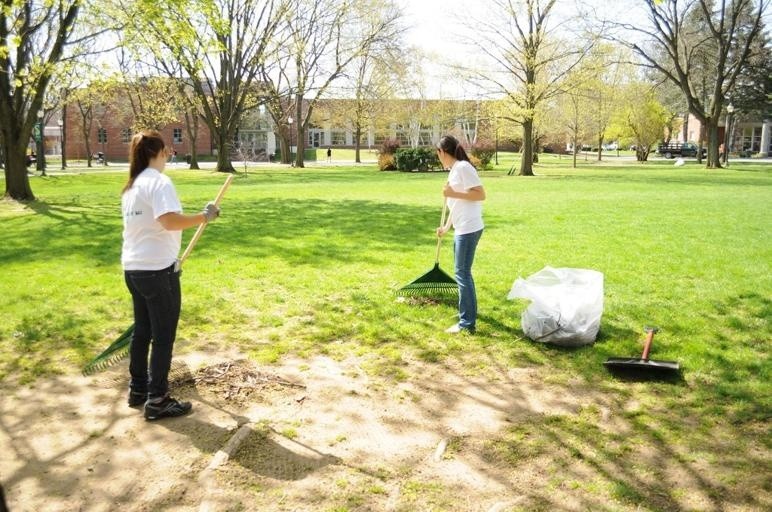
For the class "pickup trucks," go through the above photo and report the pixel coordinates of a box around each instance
[655,141,708,160]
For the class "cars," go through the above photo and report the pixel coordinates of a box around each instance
[1,151,36,168]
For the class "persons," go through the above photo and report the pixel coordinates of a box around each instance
[120,128,222,420]
[326,147,332,163]
[31,151,36,163]
[169,148,178,164]
[719,142,730,164]
[434,133,484,336]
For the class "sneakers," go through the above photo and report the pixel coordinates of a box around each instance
[143,392,193,421]
[444,324,465,335]
[126,385,149,407]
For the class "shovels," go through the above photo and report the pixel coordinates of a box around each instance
[602,326,679,371]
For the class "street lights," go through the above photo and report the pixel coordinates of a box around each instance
[288,115,294,167]
[723,100,735,167]
[36,109,47,177]
[57,119,63,155]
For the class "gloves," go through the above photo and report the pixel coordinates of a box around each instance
[203,201,220,221]
